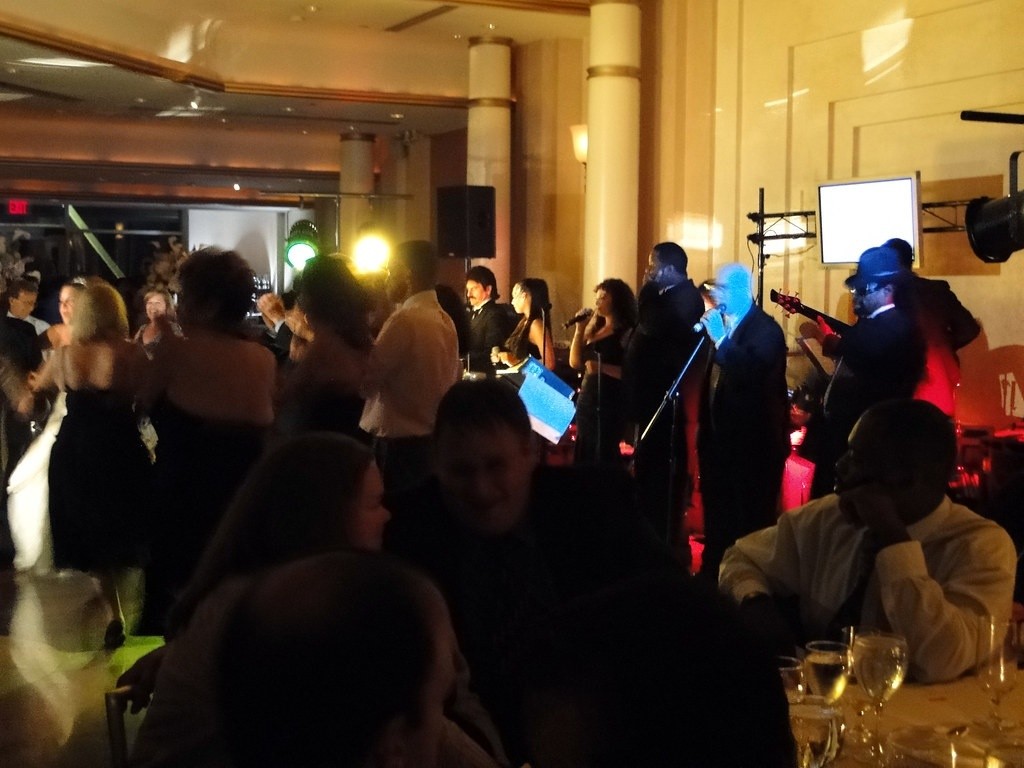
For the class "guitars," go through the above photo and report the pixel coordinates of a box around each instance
[770,289,852,336]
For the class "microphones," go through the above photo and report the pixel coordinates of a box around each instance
[691,304,726,333]
[560,309,592,330]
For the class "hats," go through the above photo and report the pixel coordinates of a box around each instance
[844,246,912,284]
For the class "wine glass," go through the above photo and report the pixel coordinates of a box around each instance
[850,632,908,768]
[973,614,1020,732]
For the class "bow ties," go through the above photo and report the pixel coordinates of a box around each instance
[471,309,480,316]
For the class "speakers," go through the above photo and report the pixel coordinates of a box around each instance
[437,183,497,260]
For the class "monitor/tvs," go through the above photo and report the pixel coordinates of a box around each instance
[817,171,925,269]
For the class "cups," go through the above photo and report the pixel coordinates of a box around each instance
[789,694,847,768]
[802,639,853,704]
[778,655,806,705]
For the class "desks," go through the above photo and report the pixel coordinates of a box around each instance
[785,666,1024,768]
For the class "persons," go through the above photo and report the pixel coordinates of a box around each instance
[0,234,1024,768]
[716,396,1018,768]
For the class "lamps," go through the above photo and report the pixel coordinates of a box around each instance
[285,219,320,272]
[345,221,398,280]
[964,188,1024,264]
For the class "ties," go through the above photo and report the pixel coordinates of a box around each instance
[827,548,879,639]
